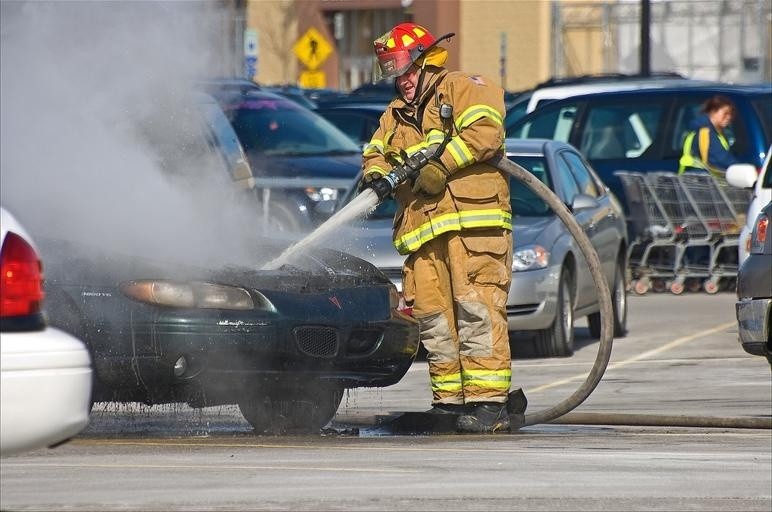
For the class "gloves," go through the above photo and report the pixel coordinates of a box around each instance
[358,172,396,200]
[410,157,450,200]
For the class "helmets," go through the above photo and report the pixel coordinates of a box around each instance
[374,23,455,82]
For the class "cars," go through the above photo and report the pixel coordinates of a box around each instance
[0,206,92,460]
[40,220,422,435]
[325,139,628,356]
[727,146,772,366]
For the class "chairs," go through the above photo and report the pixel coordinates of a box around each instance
[510,173,549,214]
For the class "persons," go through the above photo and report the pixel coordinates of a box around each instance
[676,96,744,262]
[356,21,513,434]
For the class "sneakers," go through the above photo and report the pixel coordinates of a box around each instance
[425,403,511,433]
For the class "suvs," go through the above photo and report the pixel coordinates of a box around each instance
[159,76,399,237]
